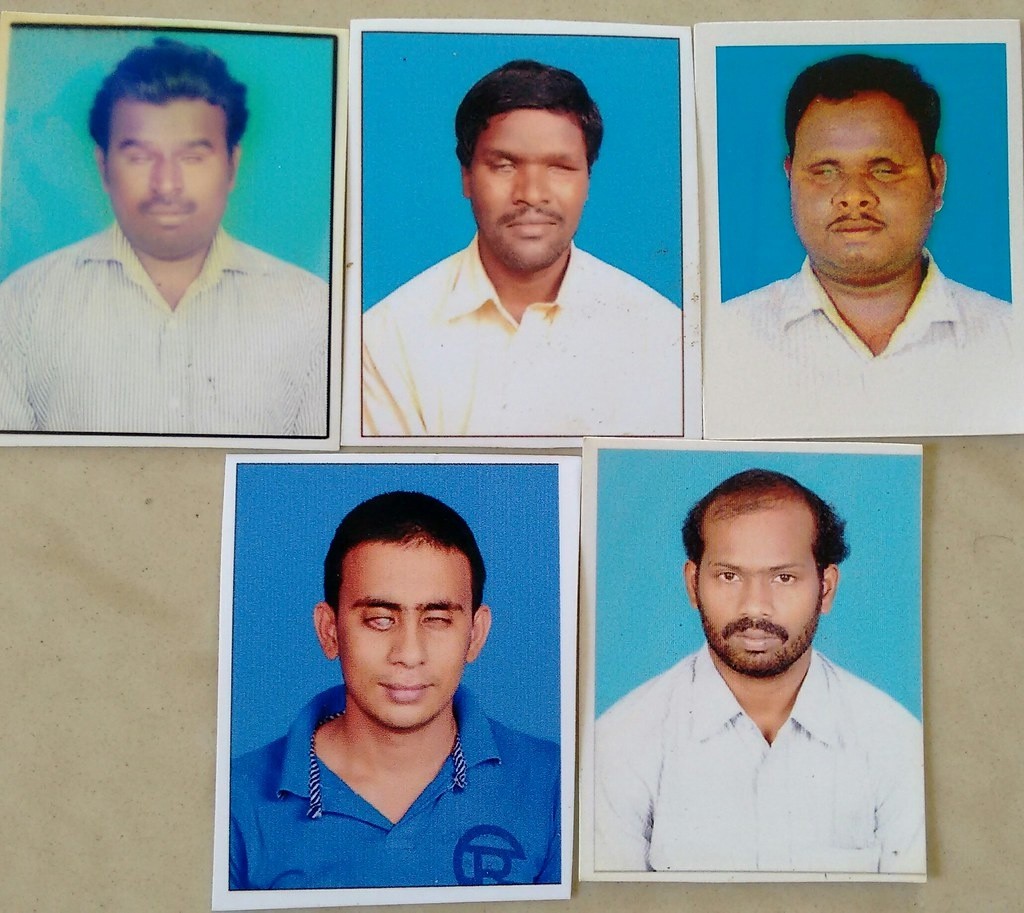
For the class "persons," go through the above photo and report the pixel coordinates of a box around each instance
[1,36,326,435]
[232,490,561,890]
[722,54,1014,436]
[593,469,926,871]
[361,58,683,437]
